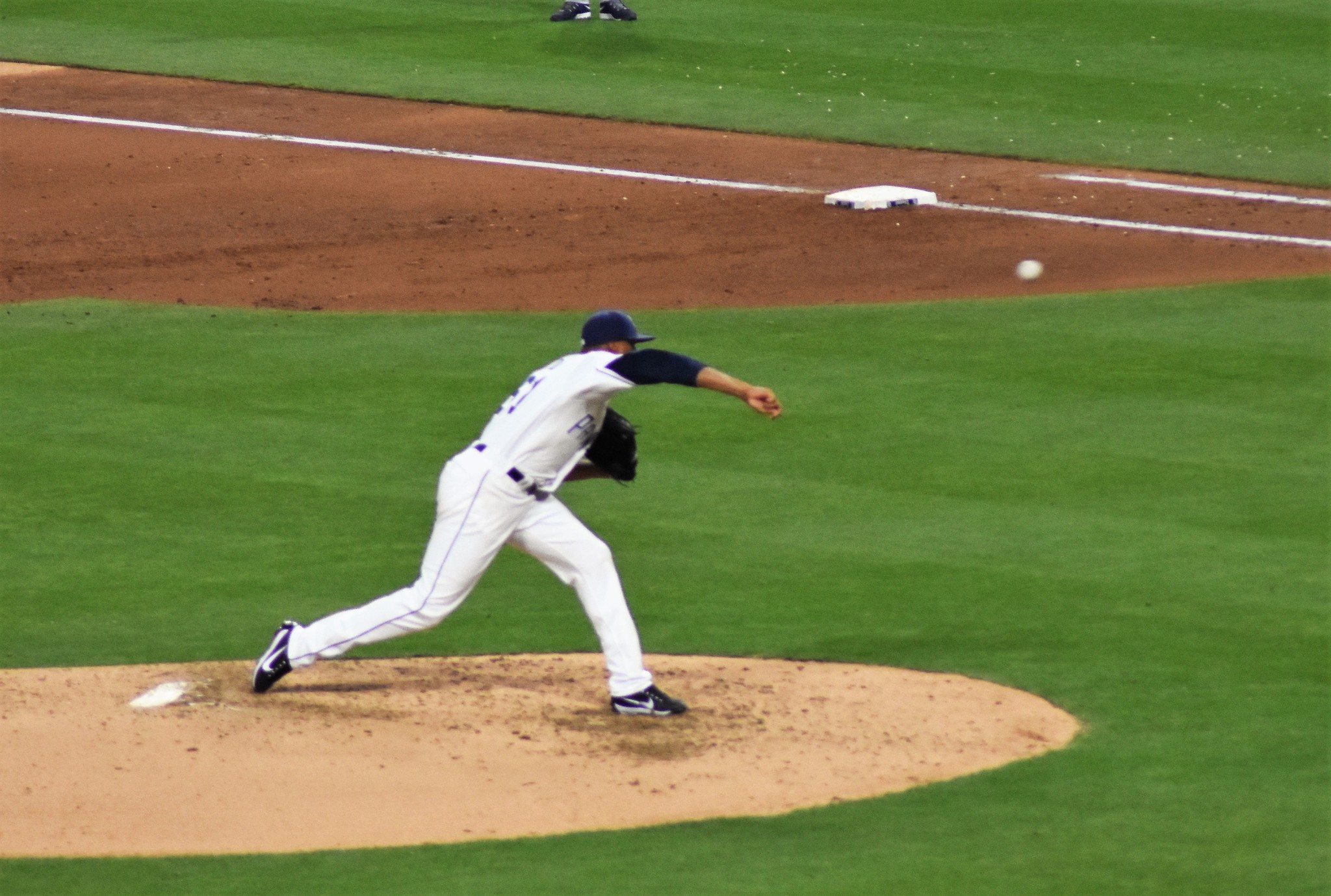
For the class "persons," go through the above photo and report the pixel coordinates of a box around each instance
[248,309,784,716]
[550,0,637,22]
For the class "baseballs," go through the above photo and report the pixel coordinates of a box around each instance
[1017,259,1042,280]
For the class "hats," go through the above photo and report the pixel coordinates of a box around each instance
[581,310,655,347]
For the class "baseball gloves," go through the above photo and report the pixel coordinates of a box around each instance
[584,407,643,489]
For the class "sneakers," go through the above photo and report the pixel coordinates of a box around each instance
[612,684,686,716]
[252,623,297,694]
[550,1,591,22]
[599,0,638,22]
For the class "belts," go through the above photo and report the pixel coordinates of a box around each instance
[475,443,538,495]
